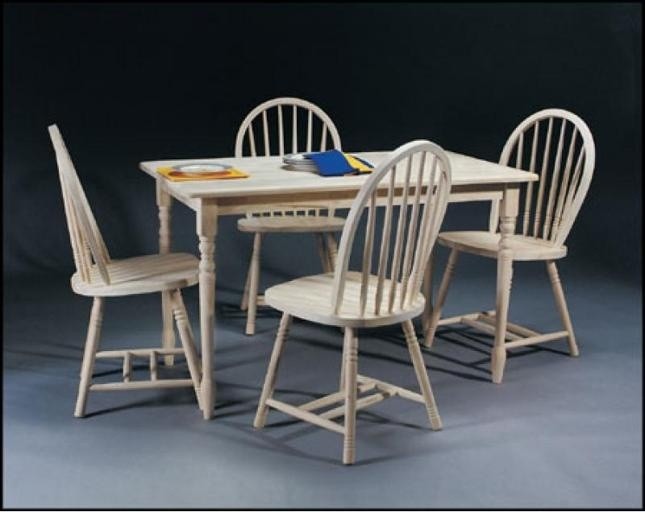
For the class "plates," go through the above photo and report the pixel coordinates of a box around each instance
[280,151,320,172]
[170,162,234,175]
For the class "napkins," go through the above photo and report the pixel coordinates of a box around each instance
[302,150,377,178]
[156,166,248,182]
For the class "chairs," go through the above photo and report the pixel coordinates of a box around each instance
[423,108,596,383]
[251,139,452,462]
[233,95,346,334]
[50,124,204,417]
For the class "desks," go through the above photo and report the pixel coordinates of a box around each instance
[139,149,538,419]
